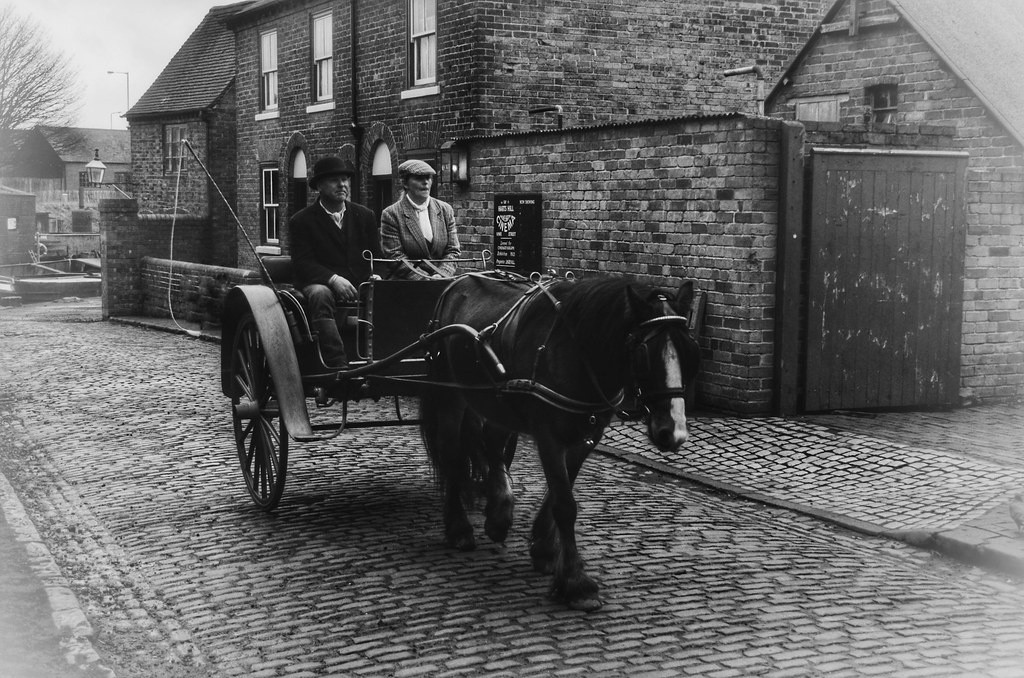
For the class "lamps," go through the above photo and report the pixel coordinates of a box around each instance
[85,148,138,187]
[439,140,467,184]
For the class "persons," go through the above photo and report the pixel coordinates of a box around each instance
[380,159,461,280]
[289,156,379,371]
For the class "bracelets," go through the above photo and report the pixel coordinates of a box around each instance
[329,275,338,286]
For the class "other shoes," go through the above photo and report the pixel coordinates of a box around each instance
[328,358,350,372]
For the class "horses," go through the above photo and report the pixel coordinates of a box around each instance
[417,266,706,612]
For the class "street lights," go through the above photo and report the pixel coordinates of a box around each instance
[111,111,121,129]
[107,71,130,112]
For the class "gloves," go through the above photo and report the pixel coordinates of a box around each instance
[368,274,382,281]
[328,274,359,305]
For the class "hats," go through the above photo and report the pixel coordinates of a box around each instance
[308,156,354,190]
[398,159,436,177]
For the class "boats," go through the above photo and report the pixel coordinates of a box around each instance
[12,270,103,304]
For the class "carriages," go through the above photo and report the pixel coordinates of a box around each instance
[221,152,700,614]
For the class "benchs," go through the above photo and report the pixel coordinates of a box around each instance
[258,255,370,363]
